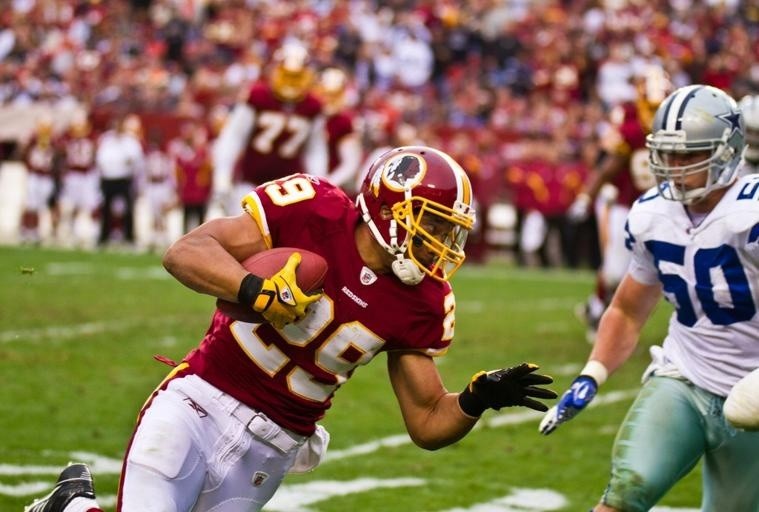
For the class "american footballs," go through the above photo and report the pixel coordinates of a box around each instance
[217,248,328,324]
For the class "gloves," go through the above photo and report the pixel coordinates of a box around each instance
[457,363,557,420]
[538,362,609,435]
[239,253,321,331]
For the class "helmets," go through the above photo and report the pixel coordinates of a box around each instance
[631,85,759,201]
[356,145,476,283]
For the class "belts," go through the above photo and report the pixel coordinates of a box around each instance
[190,372,298,455]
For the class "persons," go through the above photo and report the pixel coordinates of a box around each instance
[539,84,758,510]
[21,146,560,510]
[1,0,758,352]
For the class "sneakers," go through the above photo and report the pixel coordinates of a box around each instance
[23,461,95,511]
[574,294,605,342]
